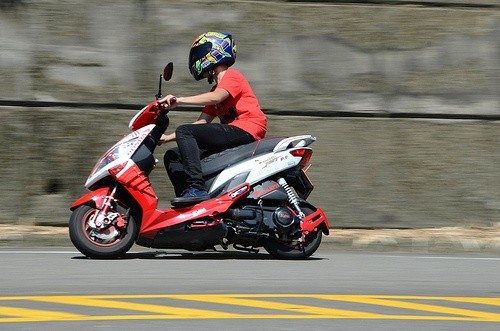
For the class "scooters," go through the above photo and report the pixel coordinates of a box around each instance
[68,60,331,261]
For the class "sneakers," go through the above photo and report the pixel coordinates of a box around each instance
[170,187,210,207]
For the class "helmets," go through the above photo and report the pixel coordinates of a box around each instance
[189,31,237,81]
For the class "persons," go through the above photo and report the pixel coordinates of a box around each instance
[158,31,268,206]
